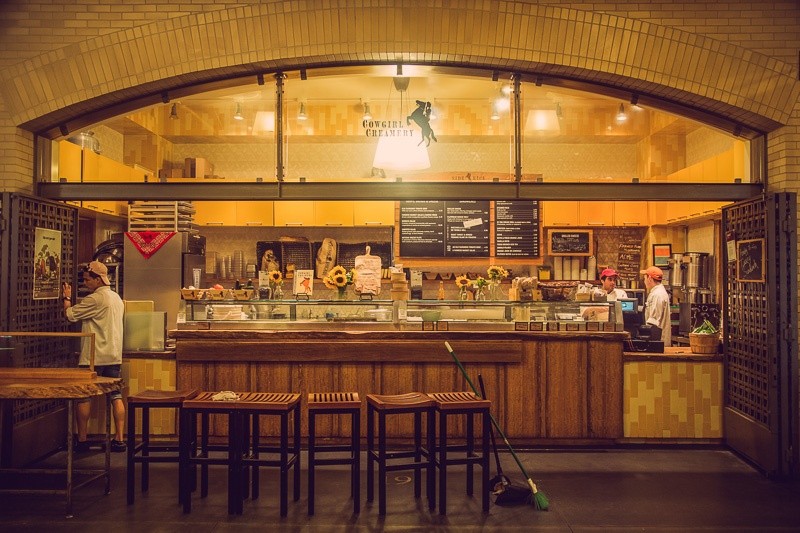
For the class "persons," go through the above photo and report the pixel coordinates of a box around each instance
[640,266,671,347]
[598,269,627,301]
[63,259,126,453]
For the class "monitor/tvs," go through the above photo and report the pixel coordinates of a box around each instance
[618,298,638,311]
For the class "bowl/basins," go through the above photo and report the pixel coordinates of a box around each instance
[420,311,440,321]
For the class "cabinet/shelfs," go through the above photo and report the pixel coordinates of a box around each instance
[127,176,395,232]
[543,143,744,228]
[58,139,157,221]
[77,261,123,299]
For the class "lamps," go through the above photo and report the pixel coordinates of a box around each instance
[616,102,627,122]
[297,103,306,119]
[362,77,431,169]
[169,103,178,120]
[232,102,245,120]
[555,103,564,120]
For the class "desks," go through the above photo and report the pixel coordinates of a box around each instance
[0,375,124,518]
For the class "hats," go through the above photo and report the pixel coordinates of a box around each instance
[87,261,111,284]
[640,266,664,280]
[601,270,618,278]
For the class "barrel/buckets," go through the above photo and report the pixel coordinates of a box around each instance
[211,305,242,320]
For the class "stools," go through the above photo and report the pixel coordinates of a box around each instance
[128,390,492,520]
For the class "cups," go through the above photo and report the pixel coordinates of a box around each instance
[219,255,233,280]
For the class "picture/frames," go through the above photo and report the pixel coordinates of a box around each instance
[737,238,766,283]
[652,243,672,267]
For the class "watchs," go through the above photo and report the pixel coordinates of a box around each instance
[62,296,71,302]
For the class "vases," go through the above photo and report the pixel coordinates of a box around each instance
[475,288,487,301]
[489,282,504,301]
[334,285,349,300]
[272,285,284,299]
[458,286,468,300]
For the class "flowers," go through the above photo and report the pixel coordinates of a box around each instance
[266,270,285,297]
[456,265,508,299]
[324,265,354,299]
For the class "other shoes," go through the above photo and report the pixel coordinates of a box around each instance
[102,439,126,451]
[65,434,89,451]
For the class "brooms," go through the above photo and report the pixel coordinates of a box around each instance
[477,372,531,507]
[443,339,552,511]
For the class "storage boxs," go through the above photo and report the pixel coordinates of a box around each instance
[159,157,214,179]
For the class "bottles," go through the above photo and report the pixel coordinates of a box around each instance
[439,281,444,300]
[275,286,283,299]
